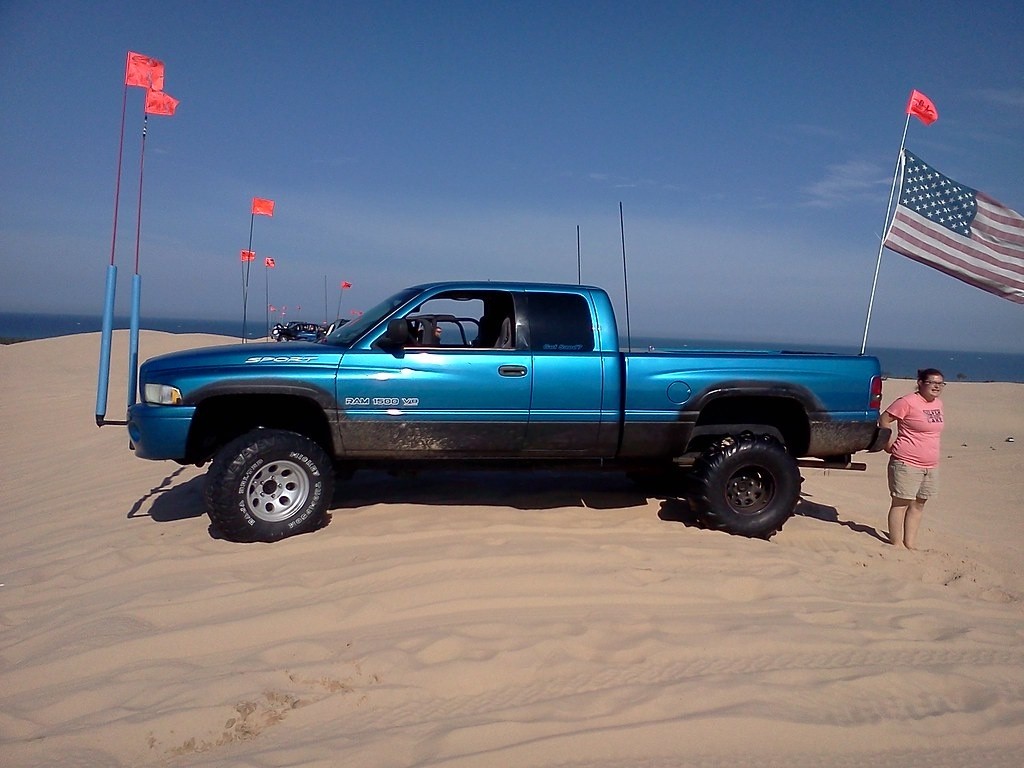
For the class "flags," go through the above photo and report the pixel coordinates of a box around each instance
[882,149,1023,307]
[241,249,363,338]
[126,51,165,91]
[906,89,938,126]
[254,198,276,217]
[146,88,180,115]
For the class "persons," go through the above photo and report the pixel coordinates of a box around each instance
[432,326,443,345]
[881,369,945,552]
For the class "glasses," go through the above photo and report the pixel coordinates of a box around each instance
[437,331,441,333]
[924,380,946,388]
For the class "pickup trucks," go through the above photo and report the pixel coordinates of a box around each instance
[324,313,480,347]
[125,281,893,543]
[270,321,323,343]
